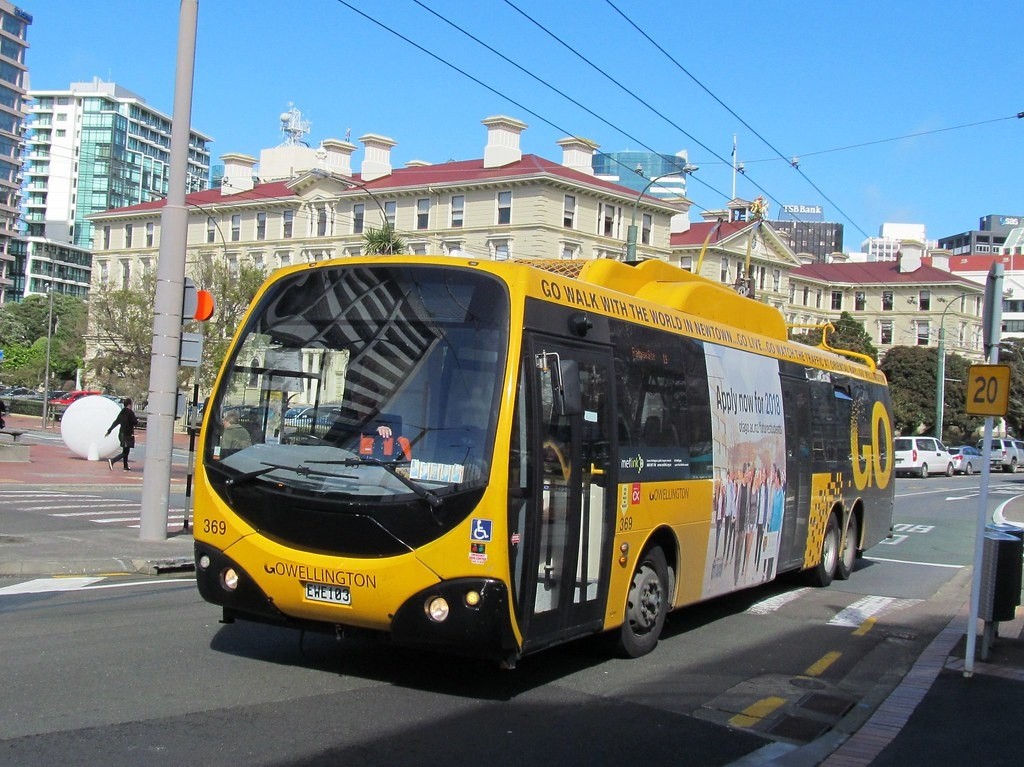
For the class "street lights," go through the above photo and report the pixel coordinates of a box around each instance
[148,187,227,381]
[309,167,394,255]
[935,294,1013,442]
[626,162,701,264]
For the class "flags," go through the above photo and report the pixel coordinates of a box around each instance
[732,142,736,156]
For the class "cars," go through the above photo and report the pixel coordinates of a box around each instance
[49,391,101,406]
[1,387,42,400]
[982,438,1024,473]
[223,405,256,417]
[114,395,131,405]
[34,390,67,401]
[949,444,988,476]
[895,436,955,480]
[326,409,341,424]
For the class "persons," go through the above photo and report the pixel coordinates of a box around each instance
[712,464,785,586]
[220,410,252,460]
[244,412,264,445]
[643,416,662,448]
[0,399,6,429]
[106,398,139,473]
[325,380,392,456]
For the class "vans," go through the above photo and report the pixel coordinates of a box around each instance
[283,406,341,425]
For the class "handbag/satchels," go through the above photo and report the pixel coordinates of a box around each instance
[0,418,5,429]
[120,436,135,448]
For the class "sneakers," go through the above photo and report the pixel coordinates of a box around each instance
[123,468,132,473]
[108,458,113,471]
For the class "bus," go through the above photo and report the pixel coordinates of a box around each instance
[191,254,896,683]
